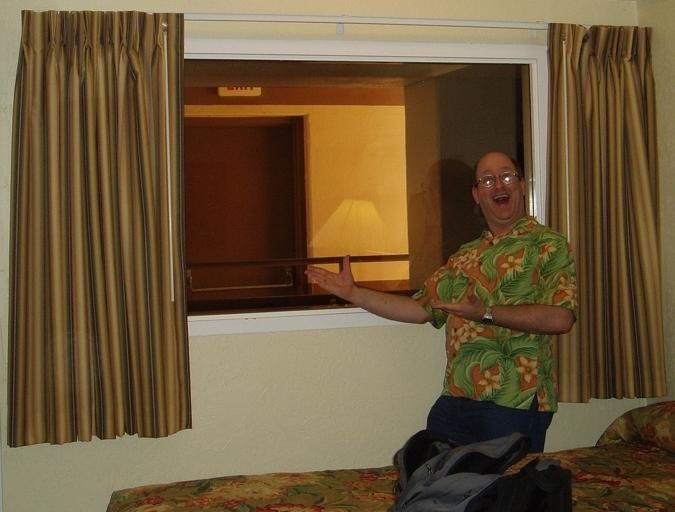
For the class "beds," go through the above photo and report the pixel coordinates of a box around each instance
[106,401,674,511]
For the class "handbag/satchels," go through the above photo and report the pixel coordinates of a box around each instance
[396,433,572,511]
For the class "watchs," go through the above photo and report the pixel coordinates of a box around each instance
[483,305,495,325]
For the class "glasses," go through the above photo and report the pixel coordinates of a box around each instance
[474,171,522,188]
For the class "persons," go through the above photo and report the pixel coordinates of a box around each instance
[302,150,581,453]
[393,157,486,299]
